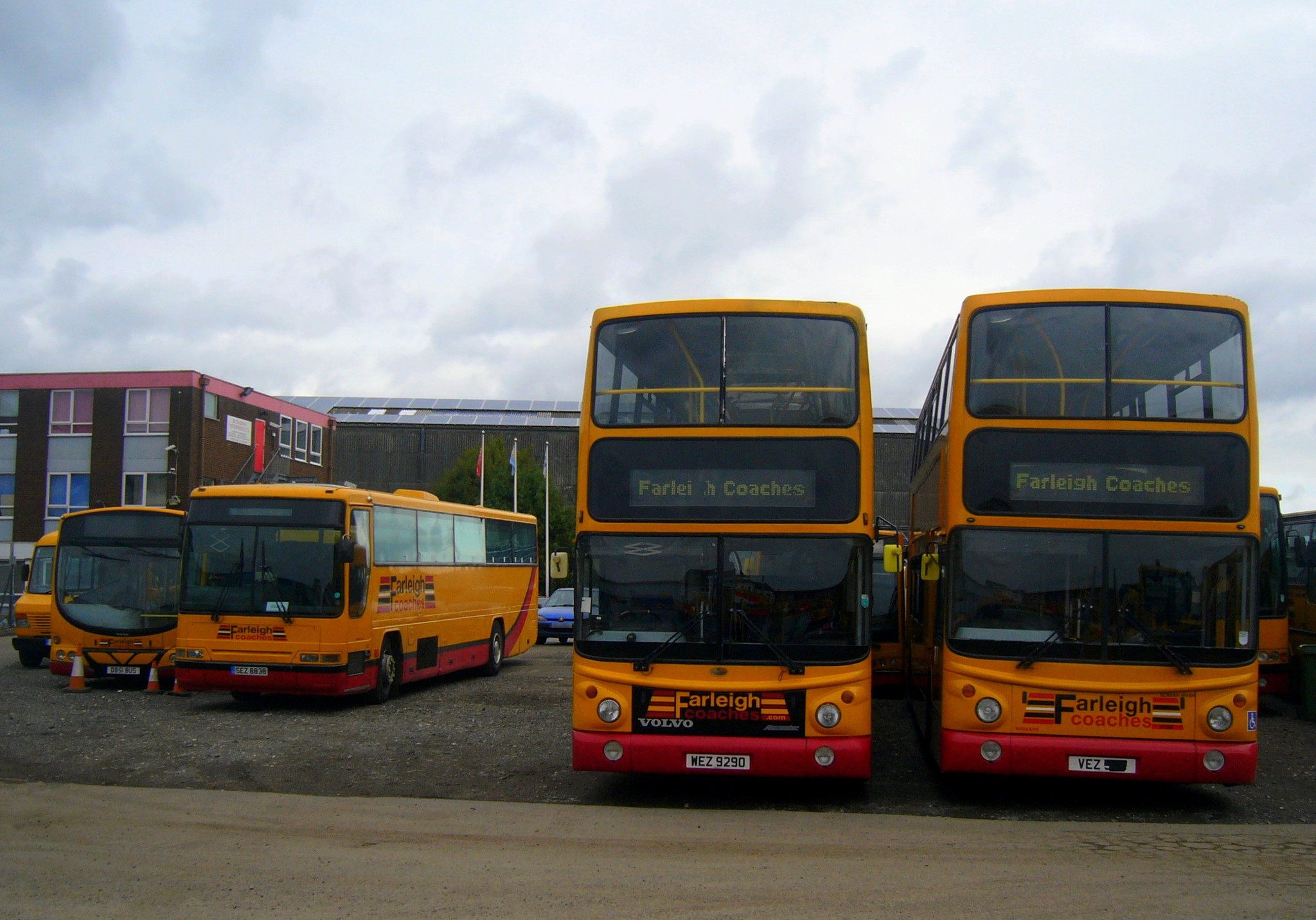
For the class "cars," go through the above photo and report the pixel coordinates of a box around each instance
[534,588,598,645]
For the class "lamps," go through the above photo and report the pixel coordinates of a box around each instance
[240,387,253,397]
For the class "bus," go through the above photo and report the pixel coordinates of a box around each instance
[1258,487,1290,717]
[51,507,189,686]
[551,296,903,785]
[175,482,540,708]
[12,530,59,667]
[904,291,1262,792]
[869,516,908,697]
[1280,510,1316,676]
